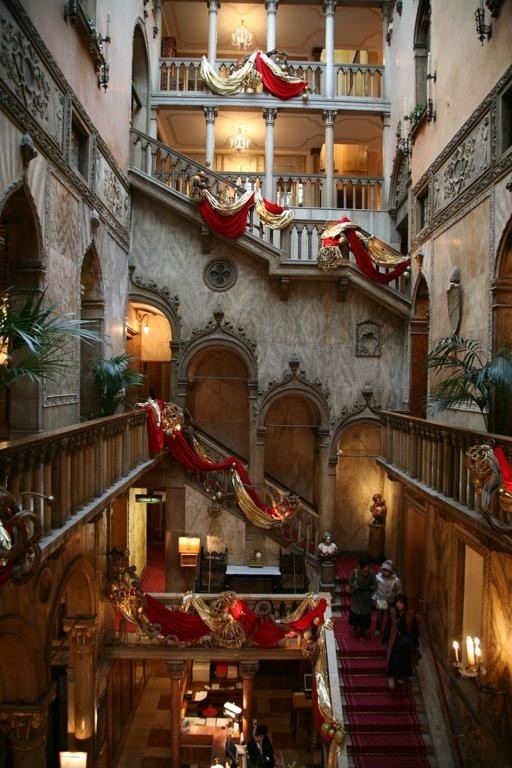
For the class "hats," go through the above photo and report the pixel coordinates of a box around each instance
[381,559,393,572]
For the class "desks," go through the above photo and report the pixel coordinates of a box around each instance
[291,693,324,752]
[225,565,282,593]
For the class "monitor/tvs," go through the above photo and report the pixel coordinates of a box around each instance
[305,674,314,693]
[227,738,237,762]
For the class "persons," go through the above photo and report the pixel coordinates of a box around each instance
[370,560,403,646]
[246,724,275,768]
[347,557,379,641]
[381,594,420,690]
[369,493,386,518]
[317,531,339,559]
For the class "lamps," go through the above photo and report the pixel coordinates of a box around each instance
[60,752,87,768]
[229,126,250,154]
[231,19,252,51]
[179,536,201,568]
[425,97,436,123]
[474,0,493,47]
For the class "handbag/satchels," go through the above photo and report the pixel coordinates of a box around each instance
[351,568,361,592]
[376,599,389,609]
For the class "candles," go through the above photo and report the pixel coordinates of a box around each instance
[453,635,481,666]
